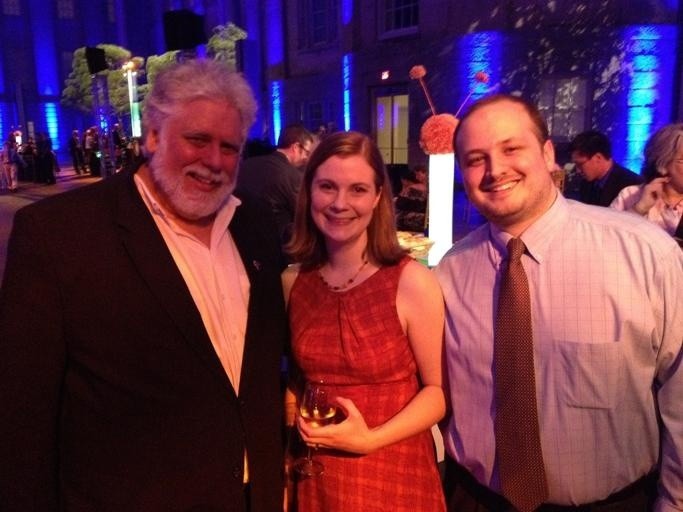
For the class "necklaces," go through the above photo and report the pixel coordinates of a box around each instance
[315,259,369,289]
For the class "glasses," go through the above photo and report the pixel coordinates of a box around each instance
[299,143,314,158]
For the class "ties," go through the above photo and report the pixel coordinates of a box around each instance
[488,236,552,511]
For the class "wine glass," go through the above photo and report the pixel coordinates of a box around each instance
[295,379,337,478]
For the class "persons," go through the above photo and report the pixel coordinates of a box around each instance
[237,121,311,265]
[271,129,455,511]
[417,95,682,511]
[609,122,682,242]
[564,127,642,212]
[1,122,130,195]
[390,162,429,232]
[0,57,308,512]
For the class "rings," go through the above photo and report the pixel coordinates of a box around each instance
[314,443,321,453]
